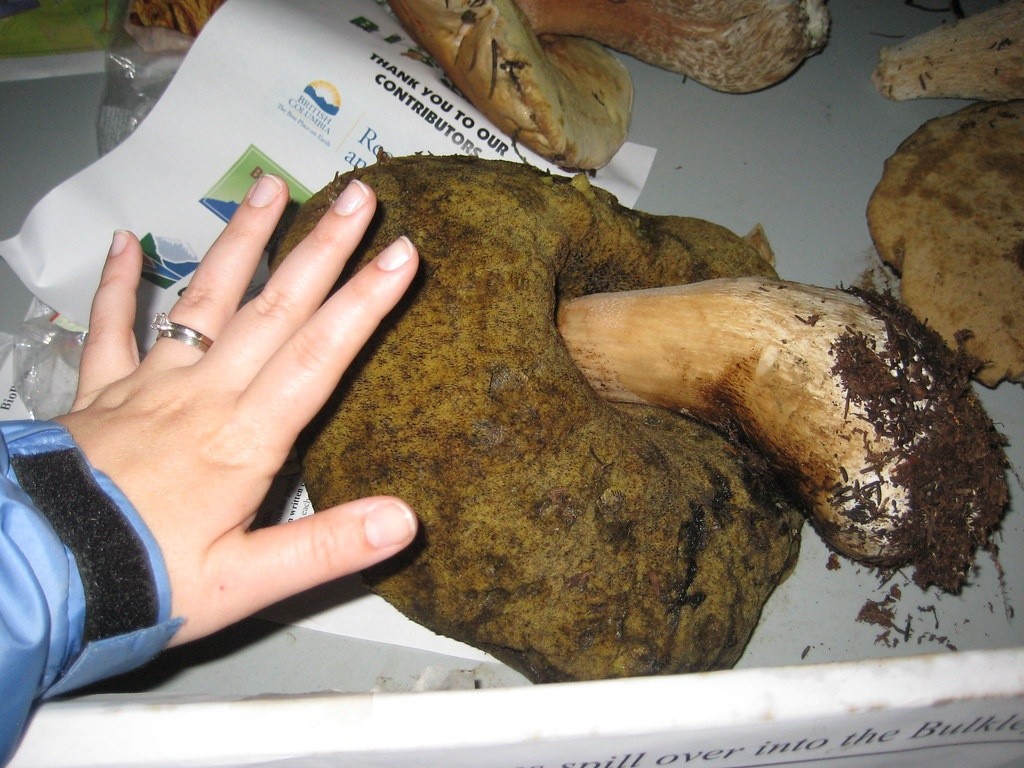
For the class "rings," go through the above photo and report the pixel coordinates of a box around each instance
[150,313,214,353]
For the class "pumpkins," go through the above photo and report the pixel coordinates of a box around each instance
[554,278,1009,562]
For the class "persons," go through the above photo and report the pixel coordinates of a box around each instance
[0,174,424,768]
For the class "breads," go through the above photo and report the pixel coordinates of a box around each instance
[511,0,830,92]
[266,154,802,683]
[385,0,633,171]
[872,0,1024,103]
[869,97,1024,389]
